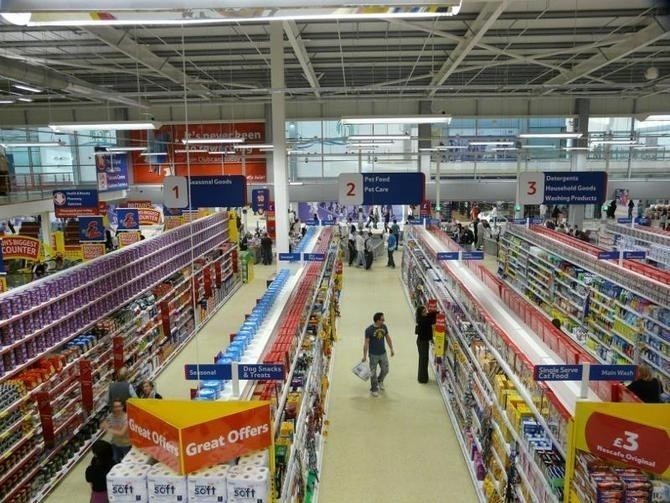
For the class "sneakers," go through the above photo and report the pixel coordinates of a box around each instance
[370,381,385,395]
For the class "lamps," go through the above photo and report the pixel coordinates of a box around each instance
[3,4,462,30]
[103,132,670,152]
[2,81,45,107]
[50,123,162,130]
[343,116,452,124]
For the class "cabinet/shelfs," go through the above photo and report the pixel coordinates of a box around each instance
[3,217,249,502]
[105,219,343,503]
[399,222,670,503]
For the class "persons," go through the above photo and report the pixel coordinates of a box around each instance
[361,312,394,396]
[107,366,138,413]
[415,304,441,384]
[237,204,419,270]
[452,202,499,250]
[137,379,162,399]
[637,199,643,215]
[85,439,116,503]
[137,230,145,240]
[625,363,664,403]
[539,202,591,242]
[628,199,635,217]
[101,398,132,464]
[552,318,561,328]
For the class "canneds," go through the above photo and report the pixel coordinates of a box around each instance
[0,407,109,503]
